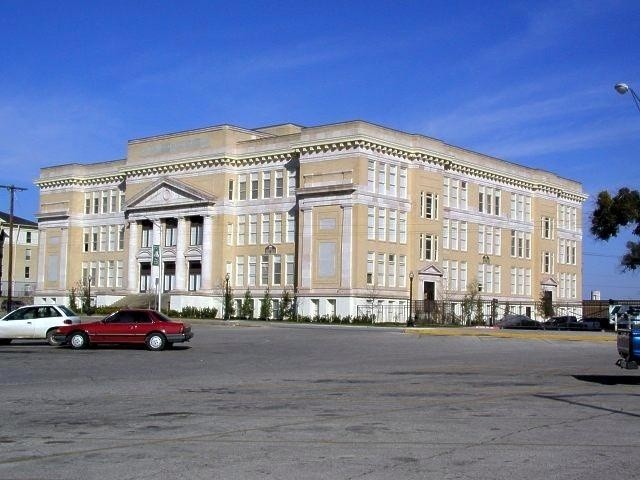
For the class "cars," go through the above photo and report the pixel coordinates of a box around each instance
[616,328,640,369]
[493,315,609,329]
[54,309,194,351]
[1,303,81,346]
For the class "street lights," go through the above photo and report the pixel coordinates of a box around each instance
[129,213,164,312]
[93,275,98,311]
[224,272,230,321]
[406,270,415,326]
